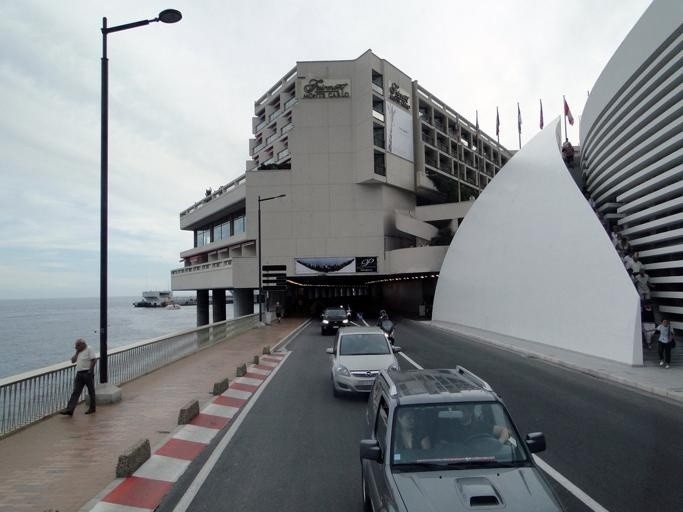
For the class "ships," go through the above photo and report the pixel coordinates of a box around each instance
[128,290,174,310]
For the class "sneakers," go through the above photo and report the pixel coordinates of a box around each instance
[660,359,670,369]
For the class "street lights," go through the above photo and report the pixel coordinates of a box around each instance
[255,193,288,323]
[93,8,183,387]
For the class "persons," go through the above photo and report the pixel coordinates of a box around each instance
[387,406,433,455]
[274,301,283,323]
[560,137,677,370]
[376,308,397,345]
[437,404,510,451]
[56,336,98,417]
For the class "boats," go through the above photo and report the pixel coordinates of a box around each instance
[183,296,239,308]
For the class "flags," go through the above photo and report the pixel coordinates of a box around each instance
[474,95,574,136]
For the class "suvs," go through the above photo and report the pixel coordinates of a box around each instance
[325,325,402,397]
[357,364,567,512]
[320,309,351,336]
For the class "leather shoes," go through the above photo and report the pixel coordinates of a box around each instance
[59,410,74,416]
[86,409,96,414]
